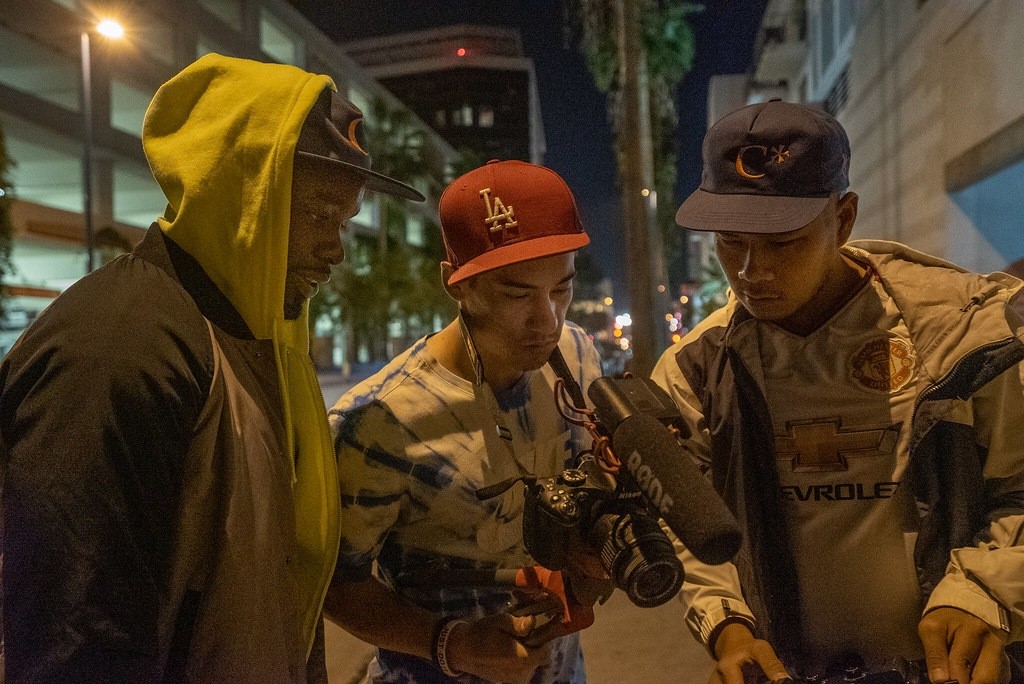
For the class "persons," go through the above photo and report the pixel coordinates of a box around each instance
[0,51,427,684]
[319,159,679,684]
[649,98,1024,684]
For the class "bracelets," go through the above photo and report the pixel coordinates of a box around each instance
[430,614,469,680]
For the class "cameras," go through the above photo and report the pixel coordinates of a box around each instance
[522,458,685,609]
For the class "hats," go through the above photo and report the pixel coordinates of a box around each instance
[438,159,590,286]
[675,98,851,235]
[297,86,425,203]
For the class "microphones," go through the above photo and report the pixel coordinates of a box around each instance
[610,412,744,567]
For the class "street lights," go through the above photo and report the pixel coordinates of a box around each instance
[78,17,122,276]
